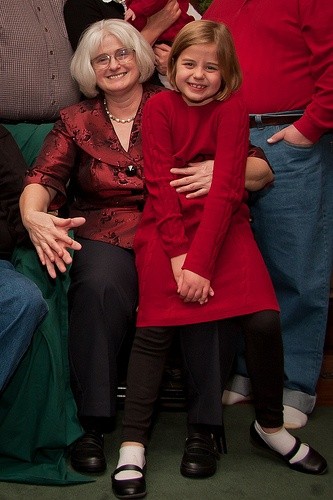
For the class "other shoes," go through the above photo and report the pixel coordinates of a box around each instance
[221,389,253,405]
[283,403,307,428]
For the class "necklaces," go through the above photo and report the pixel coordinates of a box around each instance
[104,98,136,124]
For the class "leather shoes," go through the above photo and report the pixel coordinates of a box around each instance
[249,422,328,475]
[180,432,217,479]
[111,459,147,500]
[70,430,106,474]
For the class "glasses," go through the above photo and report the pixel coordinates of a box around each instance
[91,48,135,70]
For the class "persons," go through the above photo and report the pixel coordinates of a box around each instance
[1,0,333,499]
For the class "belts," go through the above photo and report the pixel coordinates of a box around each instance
[0,116,63,126]
[249,113,303,128]
